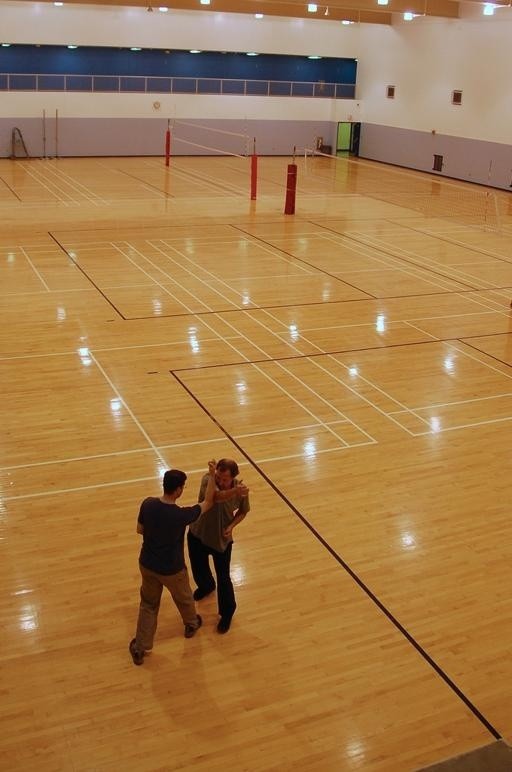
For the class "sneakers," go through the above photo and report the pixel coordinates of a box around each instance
[218,615,230,634]
[194,581,216,601]
[130,639,143,664]
[184,614,202,638]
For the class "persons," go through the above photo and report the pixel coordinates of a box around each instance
[129,456,217,665]
[186,459,250,634]
